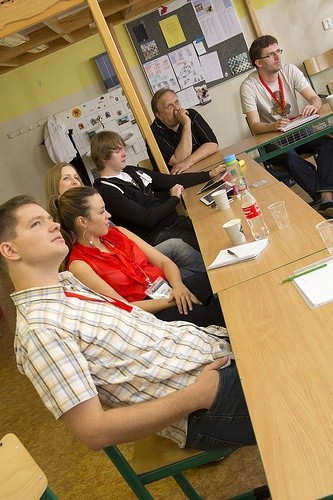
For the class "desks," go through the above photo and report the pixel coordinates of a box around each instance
[176,109,333,173]
[190,181,333,300]
[180,152,278,220]
[218,246,333,500]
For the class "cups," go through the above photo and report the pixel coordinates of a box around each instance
[222,219,247,246]
[325,94,333,111]
[268,200,290,229]
[211,189,230,211]
[315,218,333,255]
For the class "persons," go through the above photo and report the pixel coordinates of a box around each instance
[240,35,333,219]
[90,131,226,251]
[46,163,205,280]
[145,87,219,174]
[0,195,257,451]
[49,186,224,329]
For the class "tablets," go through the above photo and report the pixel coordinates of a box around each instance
[200,181,234,205]
[197,163,237,195]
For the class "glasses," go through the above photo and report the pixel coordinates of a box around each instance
[255,50,283,60]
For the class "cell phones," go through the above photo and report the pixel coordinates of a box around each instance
[211,197,234,208]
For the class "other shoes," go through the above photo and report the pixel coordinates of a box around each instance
[317,201,333,218]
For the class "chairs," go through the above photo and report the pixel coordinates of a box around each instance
[0,433,60,500]
[246,116,318,187]
[105,434,271,500]
[303,48,333,112]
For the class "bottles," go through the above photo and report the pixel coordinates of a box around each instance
[223,154,247,192]
[236,178,269,240]
